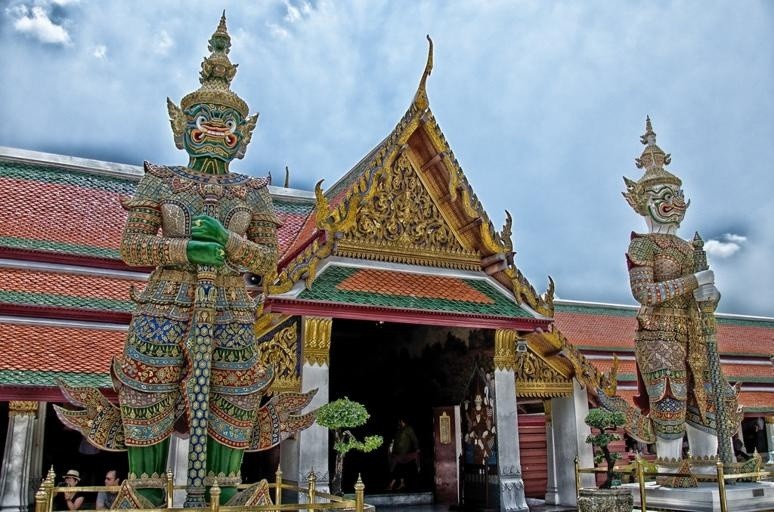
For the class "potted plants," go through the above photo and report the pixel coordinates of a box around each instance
[315,397,385,512]
[577,408,634,512]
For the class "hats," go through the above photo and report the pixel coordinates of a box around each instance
[62,469,81,481]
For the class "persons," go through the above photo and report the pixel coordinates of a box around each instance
[619,115,739,489]
[50,469,84,510]
[93,468,121,511]
[385,415,420,490]
[107,7,283,509]
